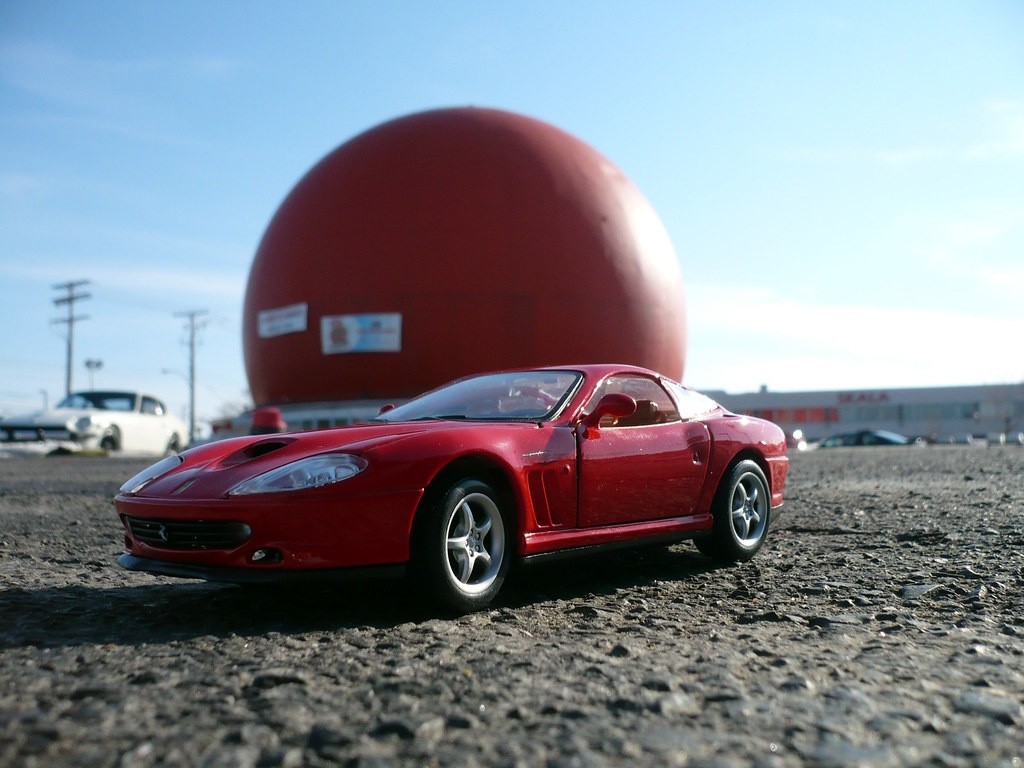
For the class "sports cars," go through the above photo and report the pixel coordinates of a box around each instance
[112,362,790,616]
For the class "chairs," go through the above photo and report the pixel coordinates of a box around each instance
[615,400,660,426]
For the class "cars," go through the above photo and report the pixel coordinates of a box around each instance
[833,427,909,448]
[0,387,191,464]
